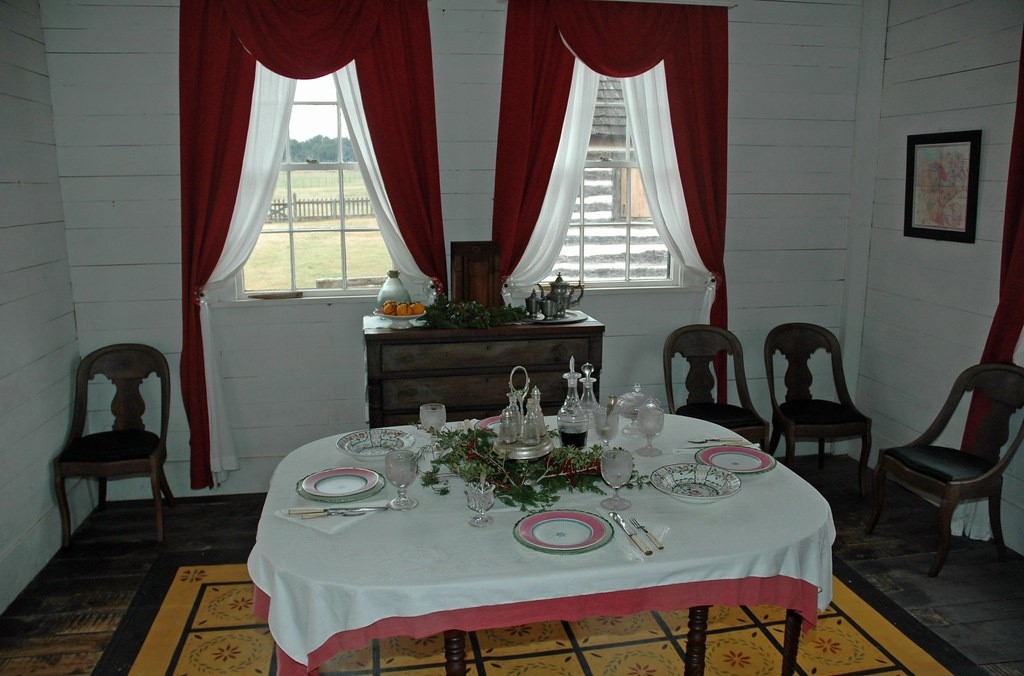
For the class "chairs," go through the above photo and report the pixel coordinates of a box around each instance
[655,323,773,458]
[864,363,1024,577]
[762,320,872,497]
[48,342,176,553]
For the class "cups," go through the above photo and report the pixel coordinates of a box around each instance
[524,296,564,320]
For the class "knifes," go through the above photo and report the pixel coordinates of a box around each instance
[288,507,387,519]
[680,441,751,449]
[608,512,653,555]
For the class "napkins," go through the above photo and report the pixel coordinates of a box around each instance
[676,435,765,454]
[274,499,390,534]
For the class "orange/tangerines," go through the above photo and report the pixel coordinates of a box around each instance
[382,300,424,316]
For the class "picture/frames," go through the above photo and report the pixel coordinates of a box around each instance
[902,129,983,246]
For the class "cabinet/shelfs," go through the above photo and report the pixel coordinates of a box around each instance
[364,306,605,429]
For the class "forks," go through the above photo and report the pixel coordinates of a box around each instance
[687,439,742,444]
[629,516,664,550]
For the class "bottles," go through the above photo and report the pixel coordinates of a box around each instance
[376,270,412,309]
[499,385,546,445]
[557,355,588,451]
[579,362,606,454]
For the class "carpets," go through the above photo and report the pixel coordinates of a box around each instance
[89,542,988,676]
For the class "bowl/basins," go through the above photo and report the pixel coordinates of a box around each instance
[650,463,741,504]
[337,429,415,461]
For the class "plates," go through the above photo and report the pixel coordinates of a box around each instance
[474,416,503,436]
[695,447,777,476]
[302,467,379,497]
[296,469,386,503]
[519,511,606,550]
[373,310,427,330]
[513,509,614,555]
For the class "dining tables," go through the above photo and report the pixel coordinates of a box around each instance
[238,412,839,676]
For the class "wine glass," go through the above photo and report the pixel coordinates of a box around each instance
[420,403,446,445]
[601,450,633,510]
[385,451,418,510]
[592,406,620,447]
[636,407,664,457]
[465,481,496,528]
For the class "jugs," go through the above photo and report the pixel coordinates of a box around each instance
[536,272,584,320]
